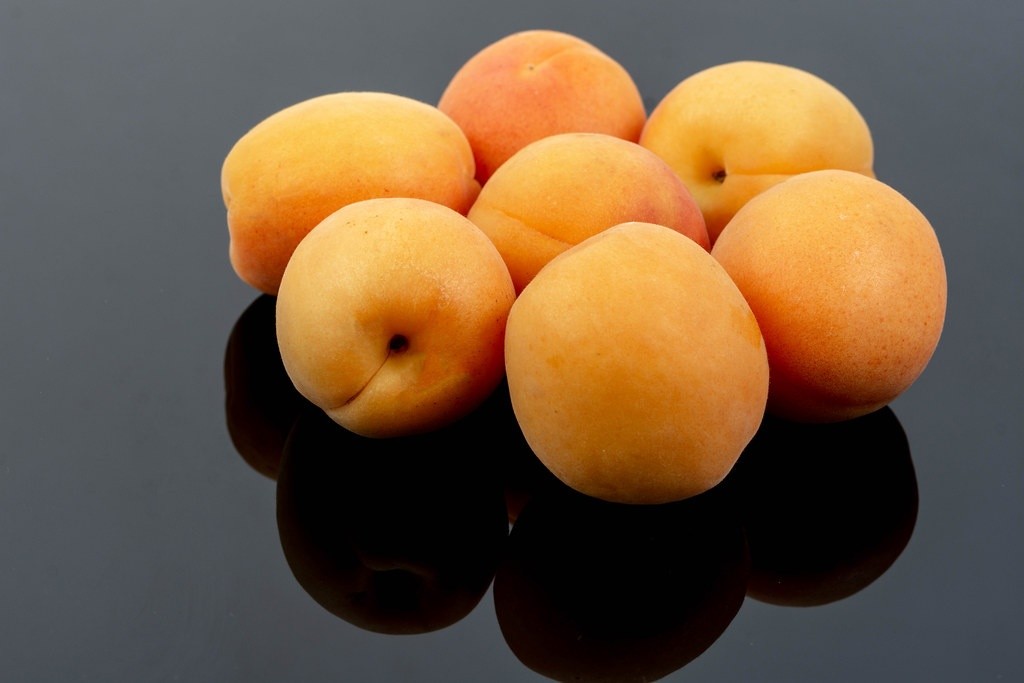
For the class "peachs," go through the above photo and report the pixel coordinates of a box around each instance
[218,29,948,505]
